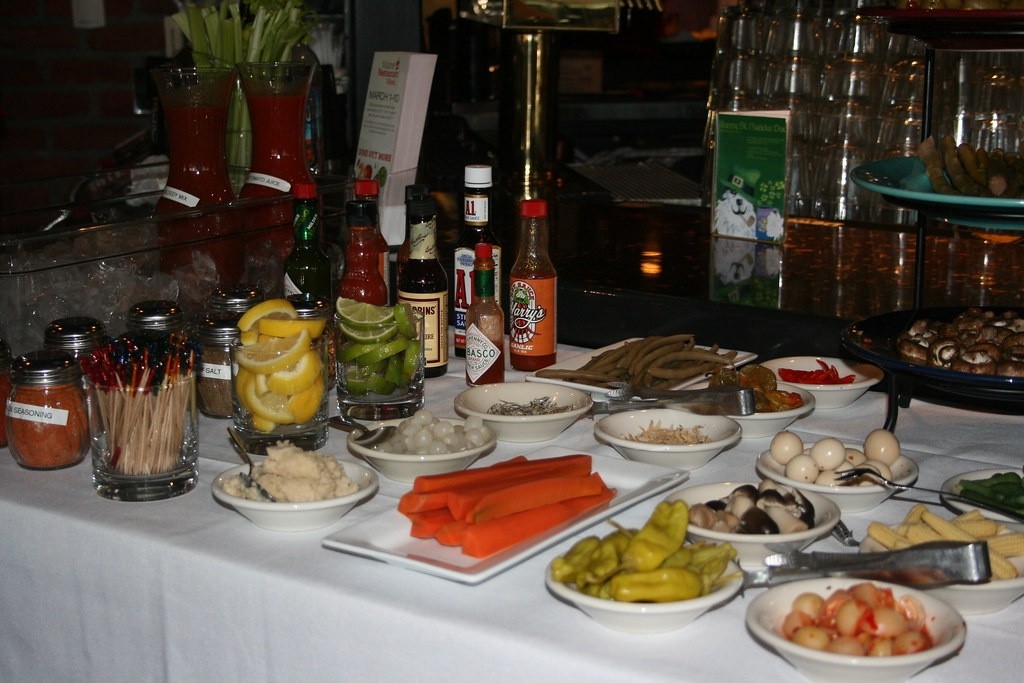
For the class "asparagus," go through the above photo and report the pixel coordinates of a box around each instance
[924,134,1024,200]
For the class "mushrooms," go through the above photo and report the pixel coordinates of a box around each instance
[898,305,1024,379]
[687,480,814,534]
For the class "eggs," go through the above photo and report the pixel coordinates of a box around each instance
[771,430,901,489]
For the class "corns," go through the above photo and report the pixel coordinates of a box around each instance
[868,504,1024,579]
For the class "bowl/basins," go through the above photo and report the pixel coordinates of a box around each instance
[544,357,1024,683]
[453,382,592,442]
[211,462,376,530]
[348,418,497,483]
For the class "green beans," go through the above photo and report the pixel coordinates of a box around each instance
[536,334,737,388]
[957,471,1024,514]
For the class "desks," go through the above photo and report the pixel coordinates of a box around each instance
[0,345,1023,682]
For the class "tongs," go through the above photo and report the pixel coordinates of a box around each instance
[739,539,989,591]
[588,385,753,418]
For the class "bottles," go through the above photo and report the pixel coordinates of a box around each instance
[285,182,332,310]
[237,62,318,198]
[152,68,235,213]
[0,283,336,470]
[508,198,556,372]
[334,166,506,386]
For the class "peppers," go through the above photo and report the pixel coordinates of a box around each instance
[551,498,747,603]
[705,365,805,413]
[778,360,858,384]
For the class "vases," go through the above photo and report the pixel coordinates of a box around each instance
[141,57,326,280]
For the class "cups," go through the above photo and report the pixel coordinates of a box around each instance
[231,333,330,454]
[703,0,1024,219]
[338,312,425,423]
[90,369,201,500]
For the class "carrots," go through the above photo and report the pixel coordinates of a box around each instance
[398,454,616,560]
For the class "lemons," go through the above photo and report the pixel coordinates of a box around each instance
[335,297,422,397]
[232,299,327,431]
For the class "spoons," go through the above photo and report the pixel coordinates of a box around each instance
[227,427,276,502]
[329,415,395,447]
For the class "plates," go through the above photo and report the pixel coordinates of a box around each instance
[526,336,759,403]
[839,305,1024,391]
[848,156,1024,230]
[318,446,689,584]
